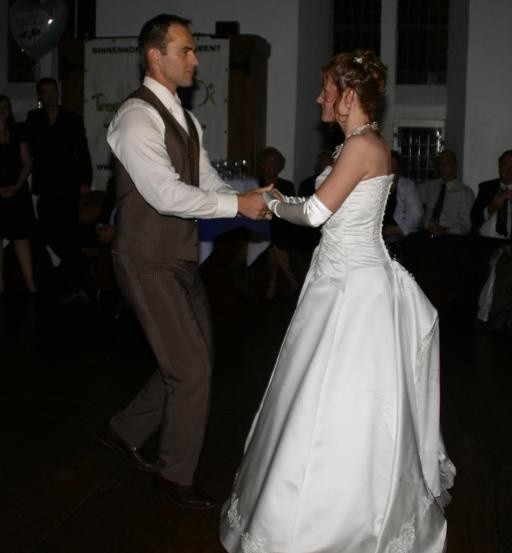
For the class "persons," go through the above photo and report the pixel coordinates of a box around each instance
[254,46,394,553]
[106,10,275,510]
[256,147,334,197]
[0,76,93,300]
[381,149,512,326]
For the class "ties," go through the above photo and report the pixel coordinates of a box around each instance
[428,184,446,233]
[496,189,507,235]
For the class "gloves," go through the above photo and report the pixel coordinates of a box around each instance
[263,188,333,228]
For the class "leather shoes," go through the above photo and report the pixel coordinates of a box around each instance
[99,424,154,472]
[152,472,218,509]
